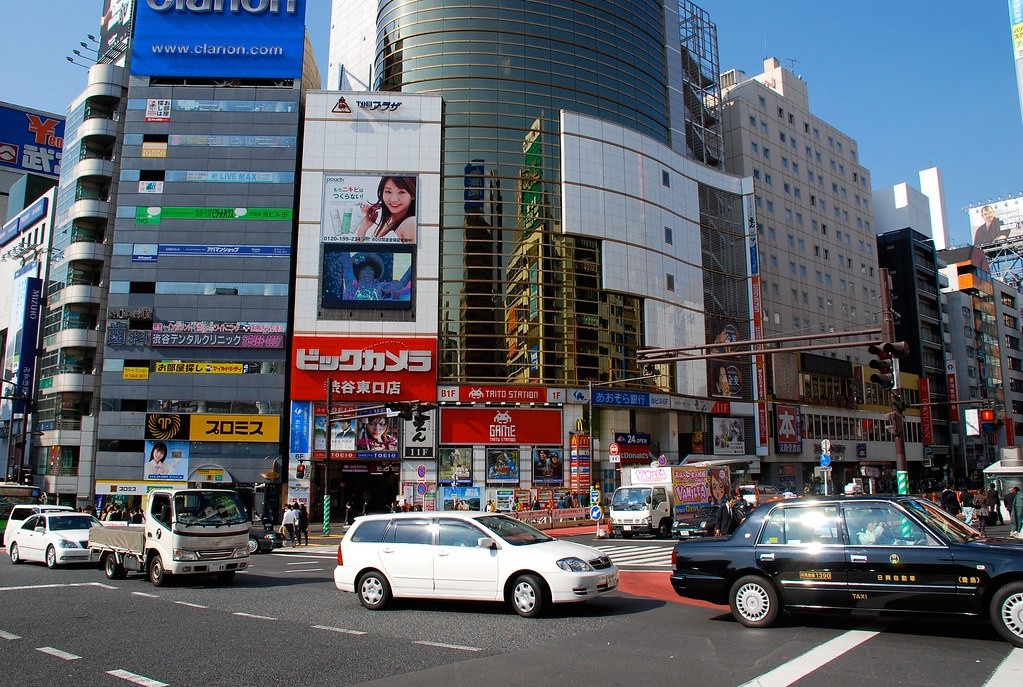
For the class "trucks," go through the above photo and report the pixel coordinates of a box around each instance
[88,489,252,588]
[609,459,754,539]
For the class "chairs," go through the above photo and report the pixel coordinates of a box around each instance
[196,497,211,518]
[50,522,59,530]
[68,519,81,528]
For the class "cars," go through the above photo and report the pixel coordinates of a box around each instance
[249,529,285,556]
[672,504,745,540]
[7,511,104,570]
[670,494,1023,649]
[332,511,620,618]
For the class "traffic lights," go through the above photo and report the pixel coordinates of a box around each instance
[414,403,434,423]
[980,408,997,435]
[296,464,306,479]
[867,341,895,391]
[384,400,413,422]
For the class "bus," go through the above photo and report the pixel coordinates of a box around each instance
[0,481,44,536]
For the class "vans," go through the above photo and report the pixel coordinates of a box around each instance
[3,504,75,547]
[738,484,785,510]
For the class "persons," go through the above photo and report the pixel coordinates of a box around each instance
[145,442,180,479]
[281,503,308,548]
[531,496,540,510]
[456,499,470,511]
[336,418,355,438]
[856,512,888,545]
[78,503,146,524]
[340,250,411,301]
[484,499,495,512]
[354,176,415,243]
[714,492,754,537]
[974,206,1011,246]
[538,451,562,478]
[558,491,591,520]
[171,497,186,521]
[394,499,423,513]
[941,482,1023,540]
[363,417,398,451]
[345,502,355,525]
[712,364,727,396]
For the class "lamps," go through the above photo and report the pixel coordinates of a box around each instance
[988,198,995,203]
[543,402,550,406]
[1018,191,1023,196]
[557,402,563,406]
[66,57,89,68]
[530,402,535,406]
[87,34,100,44]
[977,200,987,205]
[470,401,477,405]
[486,401,491,405]
[13,246,25,252]
[7,250,12,256]
[514,402,521,406]
[456,401,462,406]
[73,50,97,62]
[969,201,978,207]
[440,402,446,405]
[80,41,97,52]
[1008,193,1016,198]
[2,254,7,262]
[19,242,32,247]
[500,402,506,406]
[998,196,1007,200]
